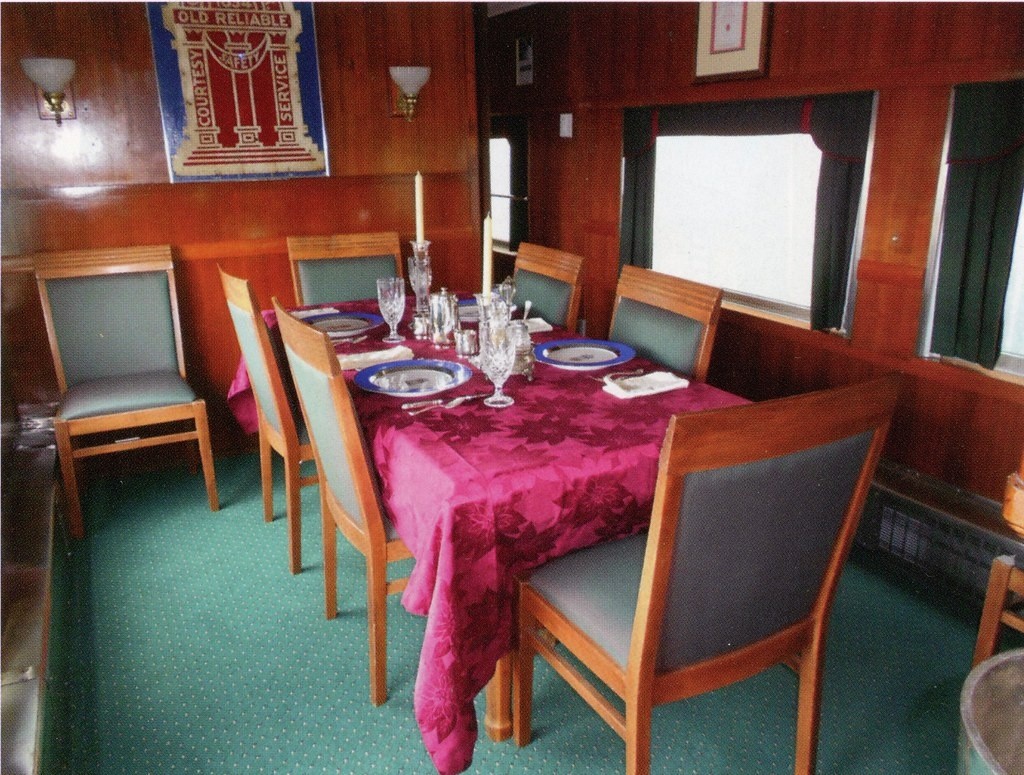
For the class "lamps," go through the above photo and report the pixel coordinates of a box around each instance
[389,66,432,122]
[20,57,76,126]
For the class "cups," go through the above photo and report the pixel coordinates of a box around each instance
[414,310,431,340]
[454,329,477,355]
[475,293,510,321]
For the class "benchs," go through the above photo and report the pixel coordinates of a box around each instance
[1,432,77,775]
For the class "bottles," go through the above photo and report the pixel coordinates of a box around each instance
[514,321,530,349]
[515,347,534,373]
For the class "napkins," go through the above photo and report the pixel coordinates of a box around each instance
[338,346,413,373]
[511,316,553,332]
[605,372,690,399]
[292,307,340,320]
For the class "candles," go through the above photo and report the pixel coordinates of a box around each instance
[414,170,425,243]
[481,213,494,295]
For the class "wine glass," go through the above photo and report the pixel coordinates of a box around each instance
[375,277,406,342]
[479,324,516,407]
[408,256,432,311]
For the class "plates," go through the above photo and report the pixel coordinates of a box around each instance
[457,299,517,324]
[534,340,636,371]
[299,312,383,338]
[353,359,472,397]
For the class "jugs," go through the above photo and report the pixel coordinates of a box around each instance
[428,288,461,349]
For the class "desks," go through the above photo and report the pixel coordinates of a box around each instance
[227,292,759,774]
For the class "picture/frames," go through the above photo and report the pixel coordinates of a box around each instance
[692,2,772,85]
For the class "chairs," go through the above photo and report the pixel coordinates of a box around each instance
[34,245,220,539]
[215,231,894,775]
[973,555,1023,665]
